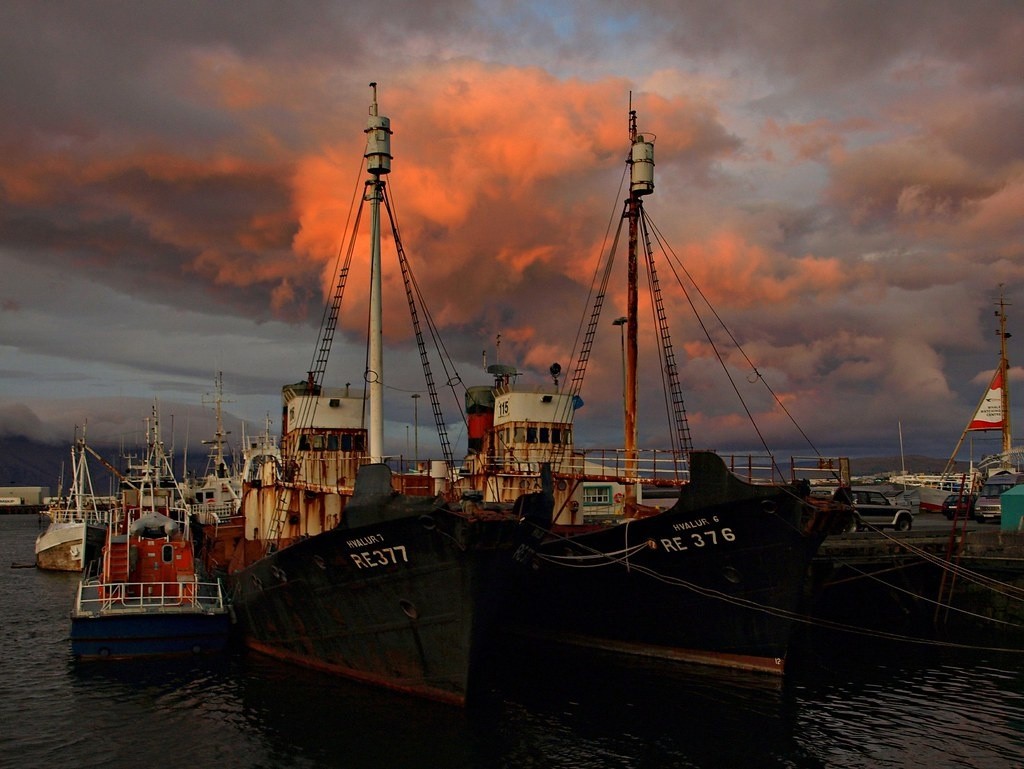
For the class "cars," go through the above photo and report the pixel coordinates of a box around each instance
[940,493,977,519]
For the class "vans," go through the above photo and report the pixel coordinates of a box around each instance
[974,472,1024,522]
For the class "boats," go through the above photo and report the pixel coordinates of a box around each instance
[426,90,858,713]
[238,73,502,731]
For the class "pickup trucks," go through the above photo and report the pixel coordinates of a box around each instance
[809,486,913,533]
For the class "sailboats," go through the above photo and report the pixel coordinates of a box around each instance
[34,371,285,666]
[919,283,1014,515]
